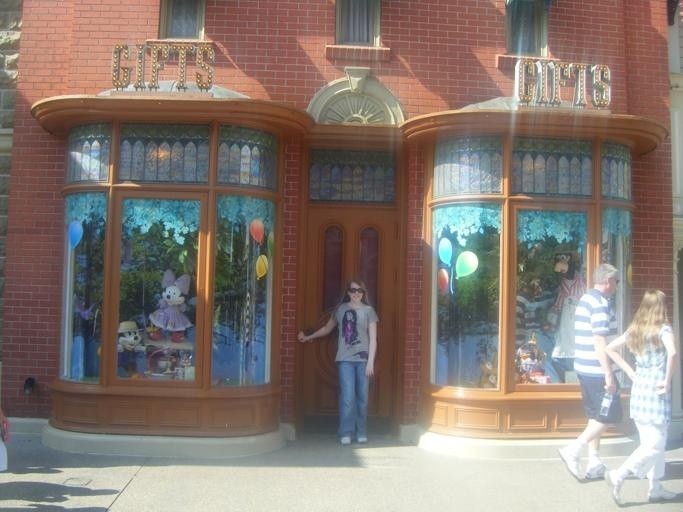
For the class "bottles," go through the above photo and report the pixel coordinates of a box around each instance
[600,392,613,417]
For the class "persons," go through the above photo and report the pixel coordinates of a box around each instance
[298,277,380,444]
[603,288,678,505]
[556,262,624,485]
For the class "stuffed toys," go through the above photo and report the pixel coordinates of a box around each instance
[147,269,194,342]
[96,320,148,382]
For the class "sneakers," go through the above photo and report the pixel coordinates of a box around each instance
[558,444,608,484]
[340,435,368,446]
[604,470,625,508]
[649,485,677,502]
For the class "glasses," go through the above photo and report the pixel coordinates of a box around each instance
[613,279,620,284]
[349,288,364,293]
